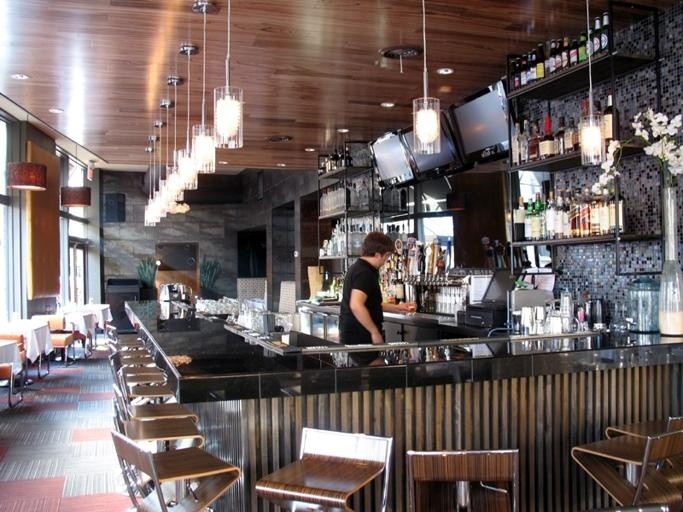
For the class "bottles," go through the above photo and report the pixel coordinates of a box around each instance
[318,144,353,175]
[322,270,342,297]
[520,337,575,352]
[329,345,450,367]
[319,180,369,216]
[379,237,451,314]
[521,291,574,337]
[323,217,407,256]
[509,12,625,241]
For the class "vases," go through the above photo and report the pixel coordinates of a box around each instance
[659,188,683,335]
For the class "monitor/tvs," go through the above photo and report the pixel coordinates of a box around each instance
[447,79,515,166]
[365,125,416,188]
[397,111,464,181]
[481,268,523,307]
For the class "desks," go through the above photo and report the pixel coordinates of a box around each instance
[0,303,113,408]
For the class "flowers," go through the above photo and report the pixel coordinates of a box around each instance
[591,107,683,196]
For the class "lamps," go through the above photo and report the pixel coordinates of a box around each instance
[5,114,47,191]
[578,0,606,166]
[193,0,216,175]
[61,144,91,207]
[215,0,244,149]
[143,44,196,228]
[411,1,443,152]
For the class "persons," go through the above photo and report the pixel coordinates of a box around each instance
[339,233,397,344]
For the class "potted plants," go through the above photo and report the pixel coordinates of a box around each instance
[199,258,221,299]
[136,255,155,301]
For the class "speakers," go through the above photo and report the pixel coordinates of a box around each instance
[107,193,126,222]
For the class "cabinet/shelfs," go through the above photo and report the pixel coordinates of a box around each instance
[106,278,142,334]
[502,33,666,275]
[317,139,387,272]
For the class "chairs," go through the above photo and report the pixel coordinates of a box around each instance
[571,431,683,511]
[112,429,241,512]
[406,448,521,512]
[605,416,682,488]
[254,427,393,512]
[102,321,202,452]
